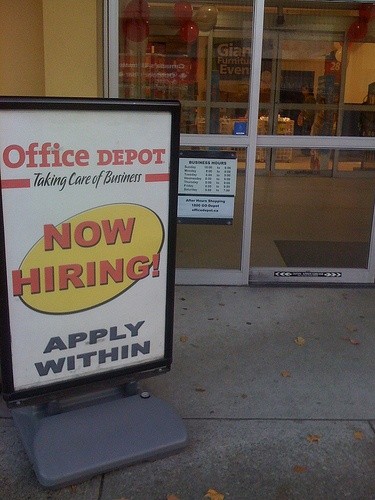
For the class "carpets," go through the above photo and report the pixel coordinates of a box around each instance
[274,238,369,268]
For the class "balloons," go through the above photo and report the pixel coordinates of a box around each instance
[123,0,150,41]
[163,0,217,46]
[169,52,196,83]
[345,3,375,43]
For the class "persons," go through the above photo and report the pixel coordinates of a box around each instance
[297,84,317,157]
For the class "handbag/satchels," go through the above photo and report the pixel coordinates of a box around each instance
[297,111,303,127]
